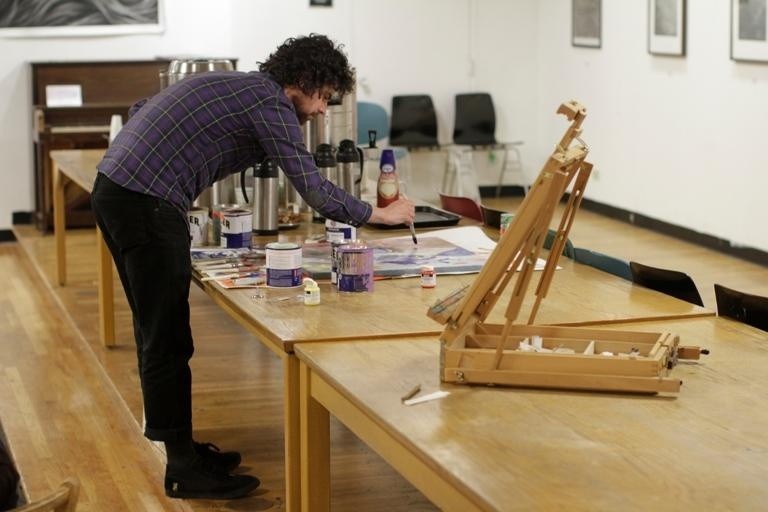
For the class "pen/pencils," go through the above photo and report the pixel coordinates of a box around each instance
[401,383,422,402]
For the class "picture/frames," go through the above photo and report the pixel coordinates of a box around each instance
[571,1,602,48]
[647,0,686,57]
[730,1,768,62]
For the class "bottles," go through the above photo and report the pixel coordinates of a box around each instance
[196,138,365,236]
[300,278,321,305]
[376,148,401,209]
[418,264,437,288]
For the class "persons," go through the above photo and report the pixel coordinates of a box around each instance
[90,35,415,500]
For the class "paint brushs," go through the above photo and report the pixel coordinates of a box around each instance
[403,195,417,245]
[194,258,259,282]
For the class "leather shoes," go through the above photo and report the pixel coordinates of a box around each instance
[164,443,260,498]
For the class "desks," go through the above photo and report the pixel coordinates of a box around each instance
[293,315,768,512]
[49,138,717,511]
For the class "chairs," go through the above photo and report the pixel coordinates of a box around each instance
[9,474,82,512]
[570,245,630,280]
[628,259,704,311]
[543,227,574,258]
[441,193,485,224]
[452,94,499,146]
[479,203,510,231]
[712,282,767,331]
[389,95,441,150]
